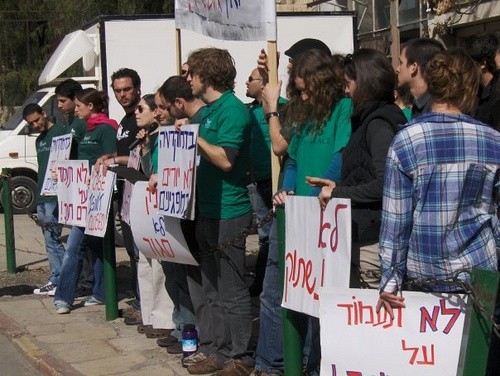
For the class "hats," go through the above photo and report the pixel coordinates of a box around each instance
[285,38,331,57]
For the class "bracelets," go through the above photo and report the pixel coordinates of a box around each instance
[113,157,116,164]
[264,112,280,121]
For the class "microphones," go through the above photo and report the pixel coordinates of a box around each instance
[128,122,159,151]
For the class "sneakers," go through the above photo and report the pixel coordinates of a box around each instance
[137,325,152,333]
[119,307,141,325]
[55,303,70,313]
[33,281,57,295]
[181,350,208,368]
[187,357,223,374]
[211,359,254,376]
[146,329,175,338]
[157,335,178,347]
[167,341,183,354]
[80,296,104,306]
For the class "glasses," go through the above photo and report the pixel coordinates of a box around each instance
[249,77,263,82]
[289,58,293,63]
[181,70,188,74]
[135,105,144,114]
[164,99,175,111]
[345,54,353,62]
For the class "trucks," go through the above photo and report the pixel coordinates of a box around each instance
[0,9,361,215]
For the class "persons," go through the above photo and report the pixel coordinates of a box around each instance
[95,67,146,298]
[56,79,94,299]
[53,88,118,313]
[23,103,66,296]
[119,33,500,376]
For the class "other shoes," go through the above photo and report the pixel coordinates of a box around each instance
[250,368,279,376]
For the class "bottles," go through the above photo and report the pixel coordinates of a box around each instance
[182,323,198,358]
